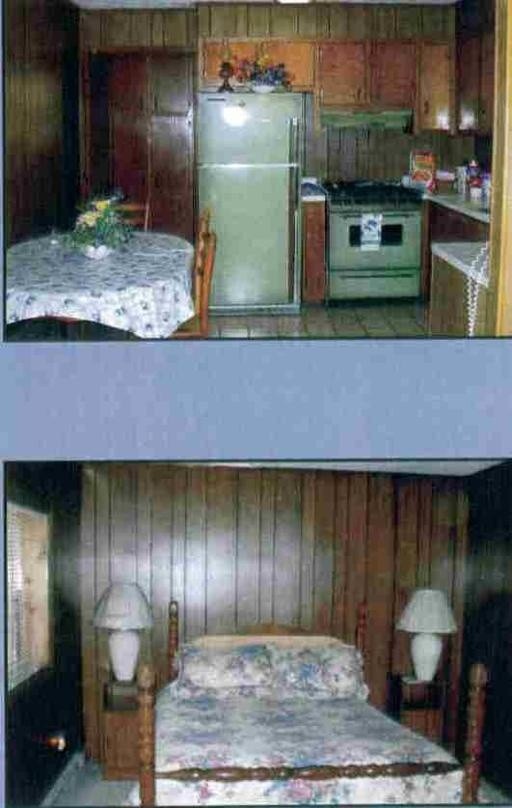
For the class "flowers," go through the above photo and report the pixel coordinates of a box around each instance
[62,195,134,252]
[236,54,296,89]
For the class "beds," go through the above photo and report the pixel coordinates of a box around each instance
[121,599,512,807]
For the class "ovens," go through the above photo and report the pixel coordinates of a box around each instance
[325,201,422,307]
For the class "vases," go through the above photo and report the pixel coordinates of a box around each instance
[80,245,112,261]
[253,83,275,94]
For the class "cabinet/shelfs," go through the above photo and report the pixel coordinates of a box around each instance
[456,33,494,137]
[425,201,490,307]
[316,40,417,113]
[417,40,453,134]
[201,37,315,93]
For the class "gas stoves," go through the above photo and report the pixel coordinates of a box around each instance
[321,178,424,205]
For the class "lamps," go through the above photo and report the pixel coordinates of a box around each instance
[396,591,457,683]
[89,580,154,685]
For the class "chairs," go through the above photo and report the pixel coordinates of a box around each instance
[116,202,153,232]
[168,209,217,340]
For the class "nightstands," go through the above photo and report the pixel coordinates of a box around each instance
[397,701,446,746]
[96,709,143,781]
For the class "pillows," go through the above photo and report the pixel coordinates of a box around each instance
[266,641,367,703]
[172,643,273,701]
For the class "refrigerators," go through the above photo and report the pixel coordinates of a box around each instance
[192,88,312,317]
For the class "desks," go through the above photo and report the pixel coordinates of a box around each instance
[5,231,198,341]
